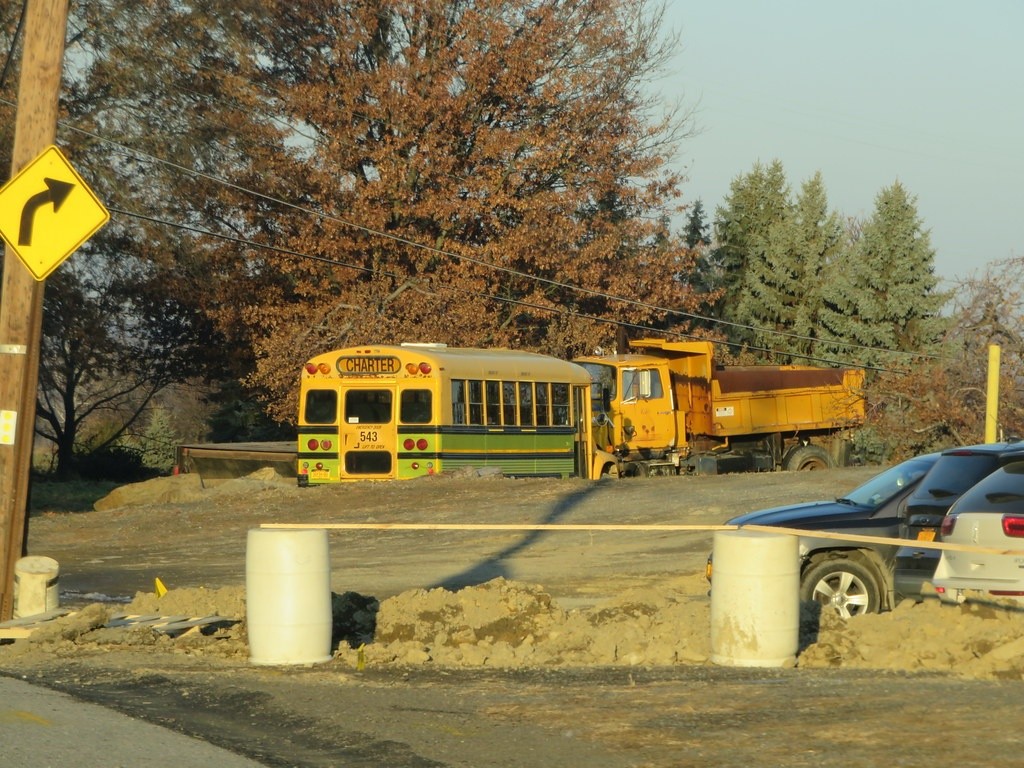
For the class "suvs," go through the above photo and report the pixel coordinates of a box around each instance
[894,442,1024,604]
[934,448,1023,610]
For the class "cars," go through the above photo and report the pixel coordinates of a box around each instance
[707,447,940,618]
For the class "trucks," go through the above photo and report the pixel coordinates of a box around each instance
[573,336,867,476]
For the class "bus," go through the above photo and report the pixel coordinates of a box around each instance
[297,339,610,490]
